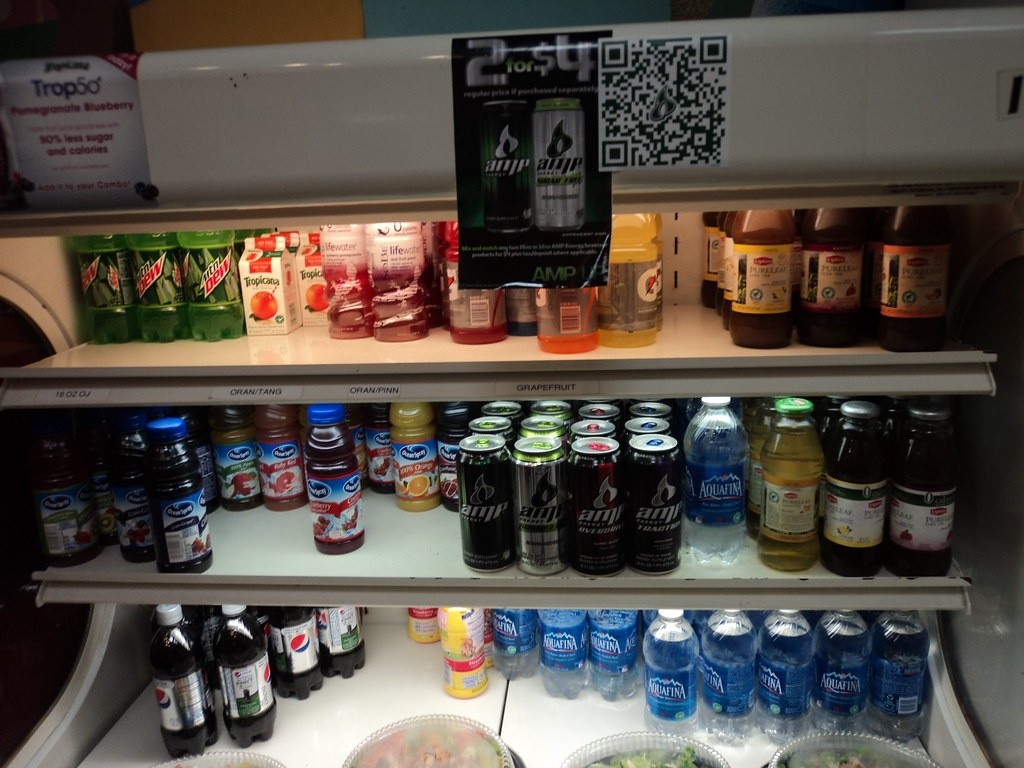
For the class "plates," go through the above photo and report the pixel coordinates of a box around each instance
[558,732,731,768]
[144,752,294,768]
[765,730,943,768]
[339,714,518,768]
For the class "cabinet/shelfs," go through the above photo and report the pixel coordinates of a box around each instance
[0,179,1024,766]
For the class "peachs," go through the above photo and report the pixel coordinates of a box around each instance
[250,291,278,320]
[306,283,330,312]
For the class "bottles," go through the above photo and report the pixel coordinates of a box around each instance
[321,216,667,356]
[684,394,956,580]
[147,605,383,760]
[404,599,929,746]
[26,401,481,576]
[68,228,278,343]
[704,198,960,353]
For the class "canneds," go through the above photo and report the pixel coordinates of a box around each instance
[456,397,685,577]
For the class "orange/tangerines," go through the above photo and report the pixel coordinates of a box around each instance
[396,475,429,497]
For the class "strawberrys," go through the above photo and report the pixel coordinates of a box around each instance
[232,472,256,494]
